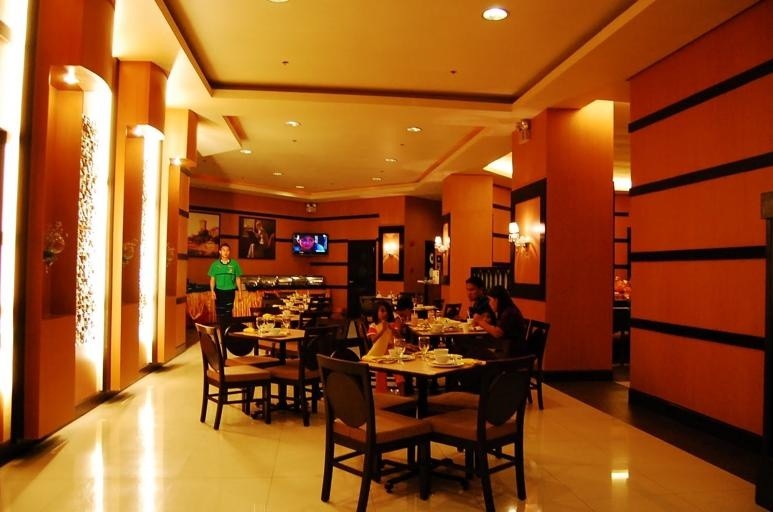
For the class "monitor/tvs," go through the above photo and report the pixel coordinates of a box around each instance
[292,232,329,255]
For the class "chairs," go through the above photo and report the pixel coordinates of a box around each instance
[317,354,433,511]
[195,294,550,460]
[330,348,418,470]
[422,355,539,510]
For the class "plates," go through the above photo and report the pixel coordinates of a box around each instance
[384,334,465,368]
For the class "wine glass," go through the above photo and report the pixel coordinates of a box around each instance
[253,290,309,336]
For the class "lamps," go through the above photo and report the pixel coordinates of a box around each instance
[433,235,450,253]
[508,222,530,248]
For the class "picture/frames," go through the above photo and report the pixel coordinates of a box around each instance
[188,209,221,258]
[238,215,276,260]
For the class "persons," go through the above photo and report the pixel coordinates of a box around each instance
[245,220,275,258]
[366,302,414,396]
[208,243,244,338]
[393,295,413,338]
[294,234,326,253]
[465,277,497,330]
[472,284,528,427]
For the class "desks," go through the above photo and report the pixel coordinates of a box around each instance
[359,354,486,499]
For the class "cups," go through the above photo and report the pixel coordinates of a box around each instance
[406,309,474,333]
[416,304,424,310]
